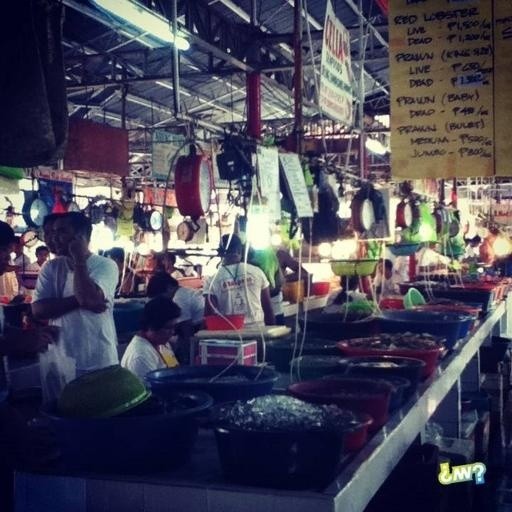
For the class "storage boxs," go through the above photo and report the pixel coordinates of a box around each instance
[199,340,257,368]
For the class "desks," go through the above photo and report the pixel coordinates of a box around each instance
[0,289,512,512]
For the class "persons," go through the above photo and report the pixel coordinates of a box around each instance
[105,247,137,297]
[29,211,120,386]
[376,258,400,298]
[119,294,183,388]
[267,242,314,321]
[241,243,283,299]
[12,236,30,270]
[146,271,205,337]
[26,246,50,273]
[420,242,469,269]
[163,253,182,279]
[0,222,19,280]
[325,272,365,307]
[41,214,68,259]
[134,253,145,269]
[464,235,481,257]
[204,235,275,328]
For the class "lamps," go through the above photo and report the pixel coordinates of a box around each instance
[61,0,191,53]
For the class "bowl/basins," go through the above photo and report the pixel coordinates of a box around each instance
[144,363,281,404]
[343,413,374,449]
[385,377,410,407]
[38,387,214,466]
[402,286,425,310]
[327,258,380,276]
[310,280,330,297]
[269,299,483,376]
[287,377,394,425]
[201,314,246,330]
[209,400,351,478]
